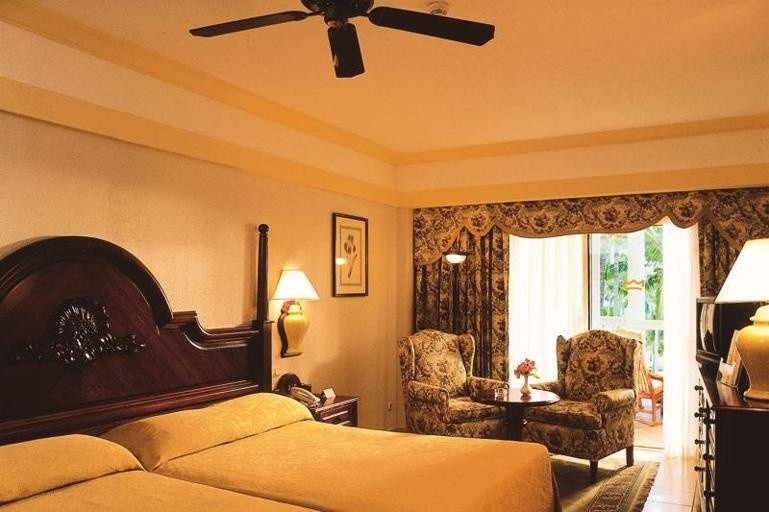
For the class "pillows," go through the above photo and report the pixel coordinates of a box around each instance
[2,435,139,505]
[103,391,313,474]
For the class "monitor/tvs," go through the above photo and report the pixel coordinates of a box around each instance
[694,297,757,368]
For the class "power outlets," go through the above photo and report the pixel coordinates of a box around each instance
[388,402,395,408]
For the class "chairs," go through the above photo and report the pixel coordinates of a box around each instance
[612,330,664,426]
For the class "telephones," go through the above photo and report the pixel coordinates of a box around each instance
[290,387,319,409]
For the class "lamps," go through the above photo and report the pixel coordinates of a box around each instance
[715,239,769,400]
[446,251,467,333]
[270,270,319,357]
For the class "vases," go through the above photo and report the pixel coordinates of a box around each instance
[521,375,532,396]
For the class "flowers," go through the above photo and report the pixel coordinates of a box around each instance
[515,358,541,380]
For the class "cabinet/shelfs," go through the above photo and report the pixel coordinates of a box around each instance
[695,366,769,512]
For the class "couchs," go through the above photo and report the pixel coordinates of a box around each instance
[397,330,510,437]
[524,331,642,480]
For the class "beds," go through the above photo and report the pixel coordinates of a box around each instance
[1,225,555,511]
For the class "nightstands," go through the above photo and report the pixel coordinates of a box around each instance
[273,373,359,429]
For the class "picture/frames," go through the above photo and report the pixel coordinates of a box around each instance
[333,213,368,298]
[721,329,744,388]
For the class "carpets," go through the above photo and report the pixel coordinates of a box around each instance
[551,449,660,511]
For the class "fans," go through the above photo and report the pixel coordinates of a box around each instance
[188,1,495,78]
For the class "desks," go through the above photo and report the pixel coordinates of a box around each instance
[471,387,560,440]
